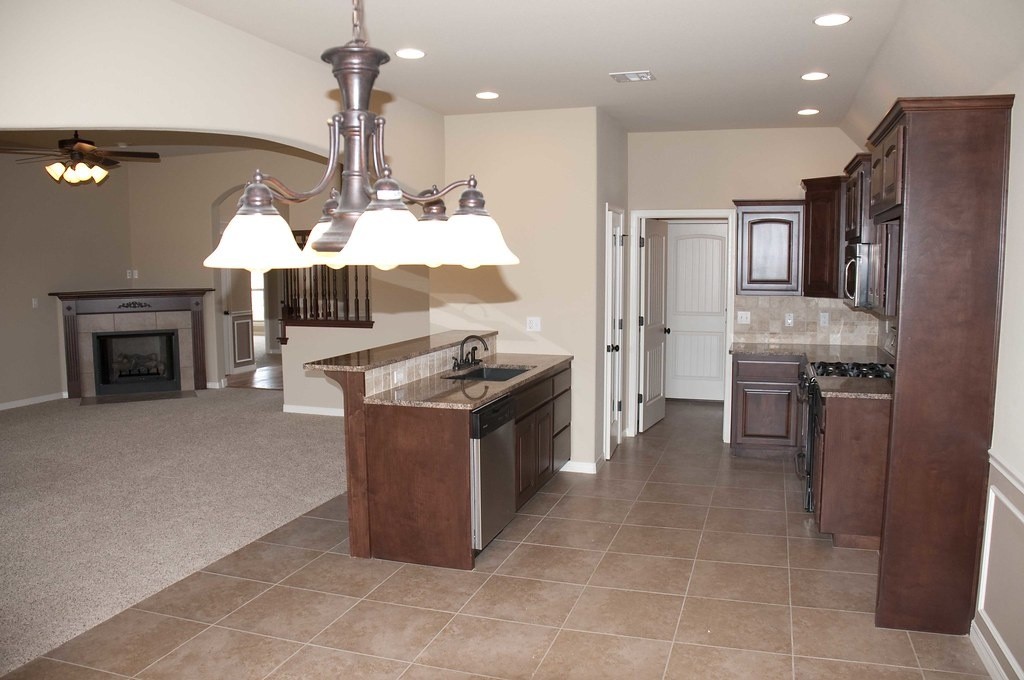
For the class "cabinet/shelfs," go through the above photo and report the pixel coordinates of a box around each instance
[729,92,1018,637]
[307,329,575,572]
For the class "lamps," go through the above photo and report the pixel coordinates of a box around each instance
[203,0,523,272]
[45,151,109,184]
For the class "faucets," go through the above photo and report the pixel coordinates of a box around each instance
[452,378,489,401]
[452,335,489,371]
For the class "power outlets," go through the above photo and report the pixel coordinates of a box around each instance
[737,311,751,325]
[820,313,829,326]
[527,317,541,332]
[785,313,793,327]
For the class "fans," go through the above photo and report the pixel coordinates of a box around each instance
[0,130,160,167]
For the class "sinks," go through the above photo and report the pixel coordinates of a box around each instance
[440,364,538,382]
